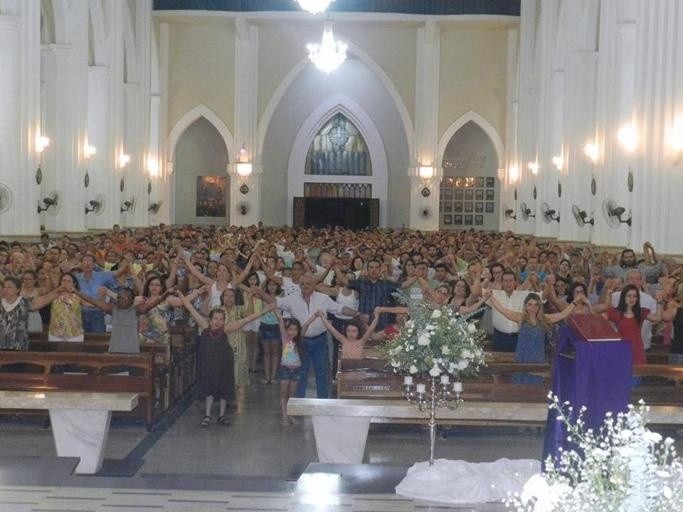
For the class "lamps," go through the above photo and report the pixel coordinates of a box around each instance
[305,15,349,74]
[416,156,435,185]
[234,144,252,178]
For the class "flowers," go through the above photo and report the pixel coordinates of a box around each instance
[500,389,683,511]
[373,290,495,380]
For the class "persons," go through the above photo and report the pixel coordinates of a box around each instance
[173,289,275,425]
[200,283,260,416]
[0,223,682,386]
[254,270,371,398]
[272,309,320,428]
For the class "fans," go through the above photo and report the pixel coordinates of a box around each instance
[601,199,630,229]
[38,190,62,216]
[85,194,106,216]
[119,196,138,215]
[521,202,535,221]
[0,183,13,214]
[572,205,594,227]
[147,200,163,214]
[541,204,560,223]
